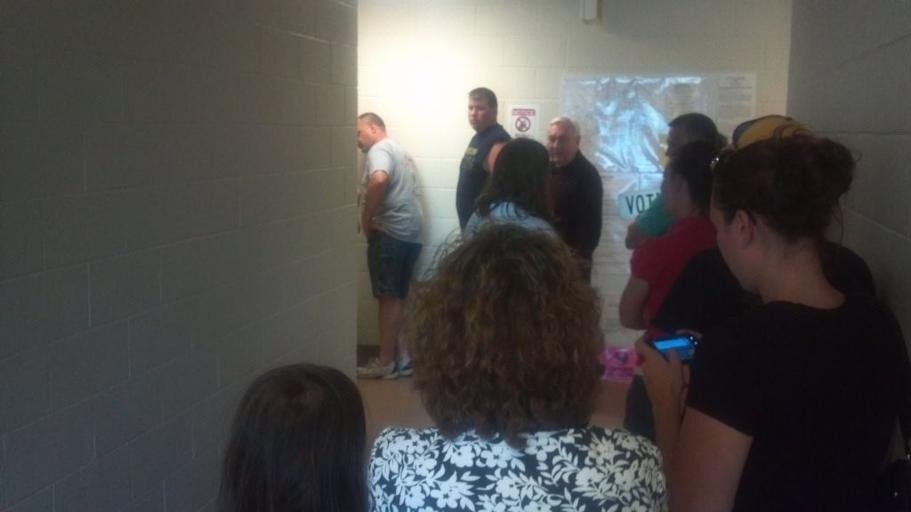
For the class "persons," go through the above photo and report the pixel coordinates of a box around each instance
[623,115,877,435]
[355,112,429,376]
[463,138,562,242]
[618,141,719,329]
[623,115,729,249]
[545,118,603,282]
[213,362,372,512]
[455,88,512,230]
[368,221,668,512]
[632,123,909,512]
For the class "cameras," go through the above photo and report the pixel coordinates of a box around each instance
[647,332,702,365]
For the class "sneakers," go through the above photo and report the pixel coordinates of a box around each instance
[394,354,415,378]
[356,358,399,380]
